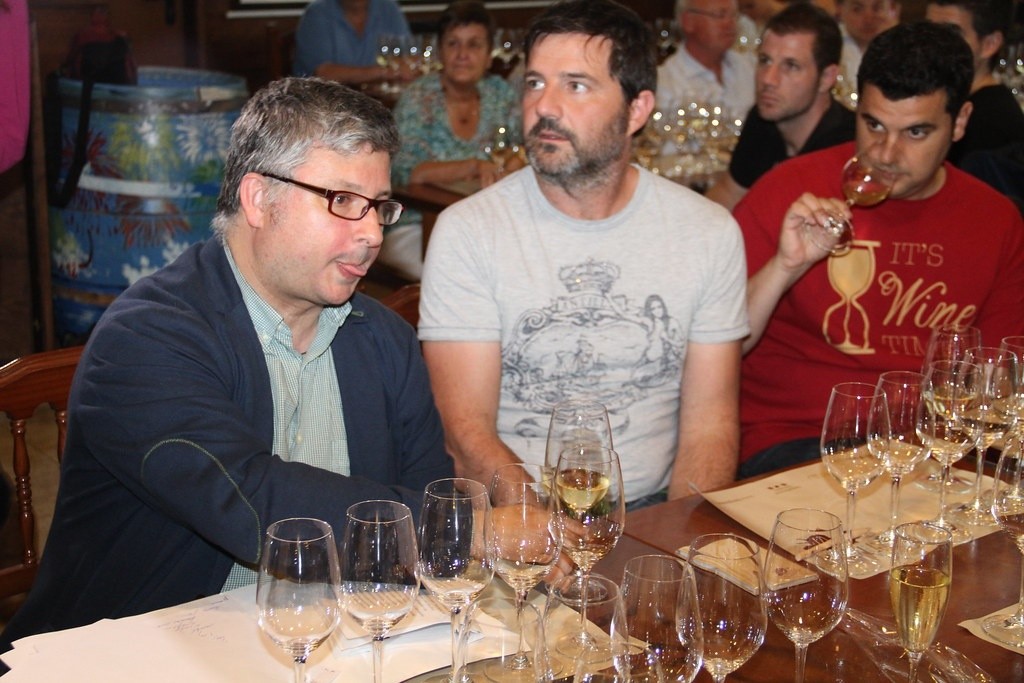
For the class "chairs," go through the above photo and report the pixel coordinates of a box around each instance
[0,347,101,635]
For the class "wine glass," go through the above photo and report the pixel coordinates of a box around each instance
[374,25,1024,255]
[254,330,1024,683]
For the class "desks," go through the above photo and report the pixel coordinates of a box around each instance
[467,429,1024,681]
[390,161,734,263]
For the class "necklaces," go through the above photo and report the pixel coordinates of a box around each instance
[460,118,468,123]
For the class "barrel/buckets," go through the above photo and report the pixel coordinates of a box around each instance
[28,63,252,349]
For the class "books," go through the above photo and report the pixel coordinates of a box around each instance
[695,537,818,595]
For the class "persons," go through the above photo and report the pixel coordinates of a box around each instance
[296,0,417,86]
[662,0,757,116]
[924,1,1024,221]
[703,0,856,213]
[418,0,751,510]
[730,23,1024,478]
[0,79,592,638]
[391,0,524,188]
[837,0,903,88]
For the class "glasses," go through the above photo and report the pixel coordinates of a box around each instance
[685,6,740,23]
[235,171,404,227]
